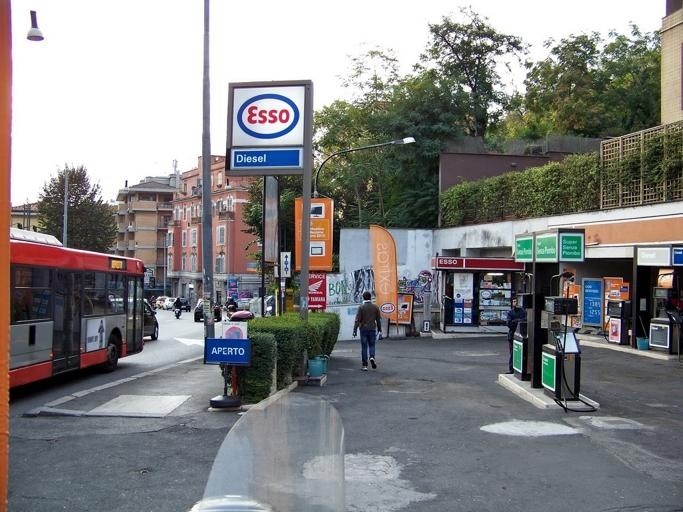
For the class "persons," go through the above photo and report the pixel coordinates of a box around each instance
[352,290,383,372]
[225,297,238,312]
[173,297,183,317]
[505,297,527,374]
[150,296,156,308]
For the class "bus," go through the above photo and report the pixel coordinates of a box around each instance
[10,228,155,390]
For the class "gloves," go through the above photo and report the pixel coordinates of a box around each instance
[378,329,382,332]
[353,331,357,337]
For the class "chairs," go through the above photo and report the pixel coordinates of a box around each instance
[85,296,94,313]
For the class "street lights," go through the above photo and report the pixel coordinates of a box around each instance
[312,137,416,198]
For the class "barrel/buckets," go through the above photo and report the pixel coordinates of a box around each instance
[315,356,329,374]
[636,337,649,350]
[307,359,323,377]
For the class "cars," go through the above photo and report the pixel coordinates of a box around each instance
[111,297,158,339]
[235,298,252,311]
[193,300,222,322]
[156,296,191,313]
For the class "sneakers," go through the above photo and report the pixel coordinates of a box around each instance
[361,366,368,371]
[369,356,377,368]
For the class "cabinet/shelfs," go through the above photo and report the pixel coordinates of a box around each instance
[478,271,513,326]
